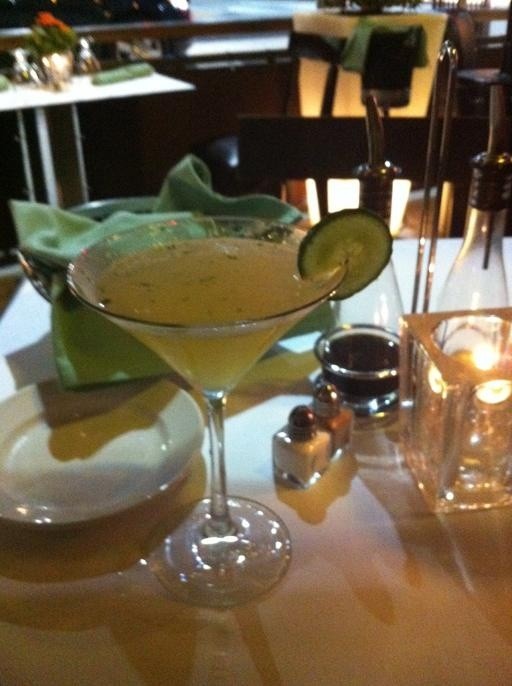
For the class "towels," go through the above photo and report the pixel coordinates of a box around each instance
[11,156,336,388]
[93,63,155,85]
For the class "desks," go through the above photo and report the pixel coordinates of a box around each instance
[0,70,197,212]
[0,235,512,686]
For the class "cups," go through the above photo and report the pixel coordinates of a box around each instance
[312,325,412,404]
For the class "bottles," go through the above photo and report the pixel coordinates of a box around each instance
[271,383,353,486]
[327,163,406,319]
[436,152,507,318]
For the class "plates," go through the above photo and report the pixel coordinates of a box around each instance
[0,377,205,532]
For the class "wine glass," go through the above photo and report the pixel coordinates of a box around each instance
[67,217,347,611]
